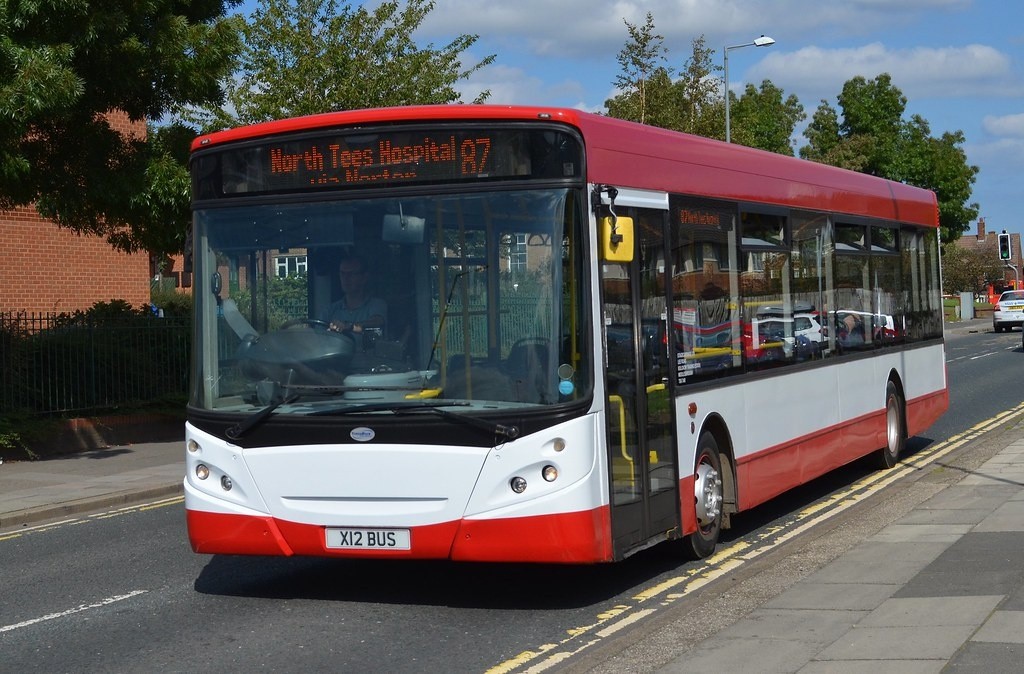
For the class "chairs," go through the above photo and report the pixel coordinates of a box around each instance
[505,336,550,378]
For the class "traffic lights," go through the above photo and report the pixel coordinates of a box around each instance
[998,234,1011,261]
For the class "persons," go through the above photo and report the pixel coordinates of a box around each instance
[317,255,388,341]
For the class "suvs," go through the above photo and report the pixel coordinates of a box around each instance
[754,303,836,356]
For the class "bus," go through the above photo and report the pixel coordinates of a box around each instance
[989,280,1024,304]
[184,102,948,565]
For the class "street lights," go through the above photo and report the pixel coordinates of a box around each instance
[722,34,776,144]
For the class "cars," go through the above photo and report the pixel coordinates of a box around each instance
[993,290,1024,332]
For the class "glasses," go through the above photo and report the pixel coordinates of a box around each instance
[338,271,357,278]
